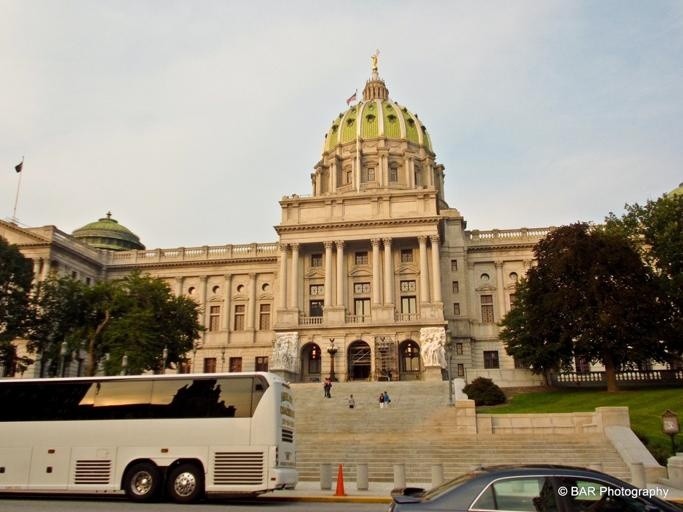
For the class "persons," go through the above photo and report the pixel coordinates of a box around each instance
[270,332,297,370]
[322,378,332,398]
[368,45,381,69]
[383,391,390,409]
[420,328,447,369]
[561,479,609,511]
[378,392,384,409]
[347,394,355,408]
[322,377,328,397]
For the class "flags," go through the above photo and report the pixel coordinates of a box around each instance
[345,92,356,106]
[13,157,24,173]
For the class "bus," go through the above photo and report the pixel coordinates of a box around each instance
[0,372,298,504]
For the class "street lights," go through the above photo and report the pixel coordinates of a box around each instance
[324,338,337,382]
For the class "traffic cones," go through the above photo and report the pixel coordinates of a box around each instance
[332,464,346,496]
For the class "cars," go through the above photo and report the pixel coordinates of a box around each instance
[386,465,681,511]
